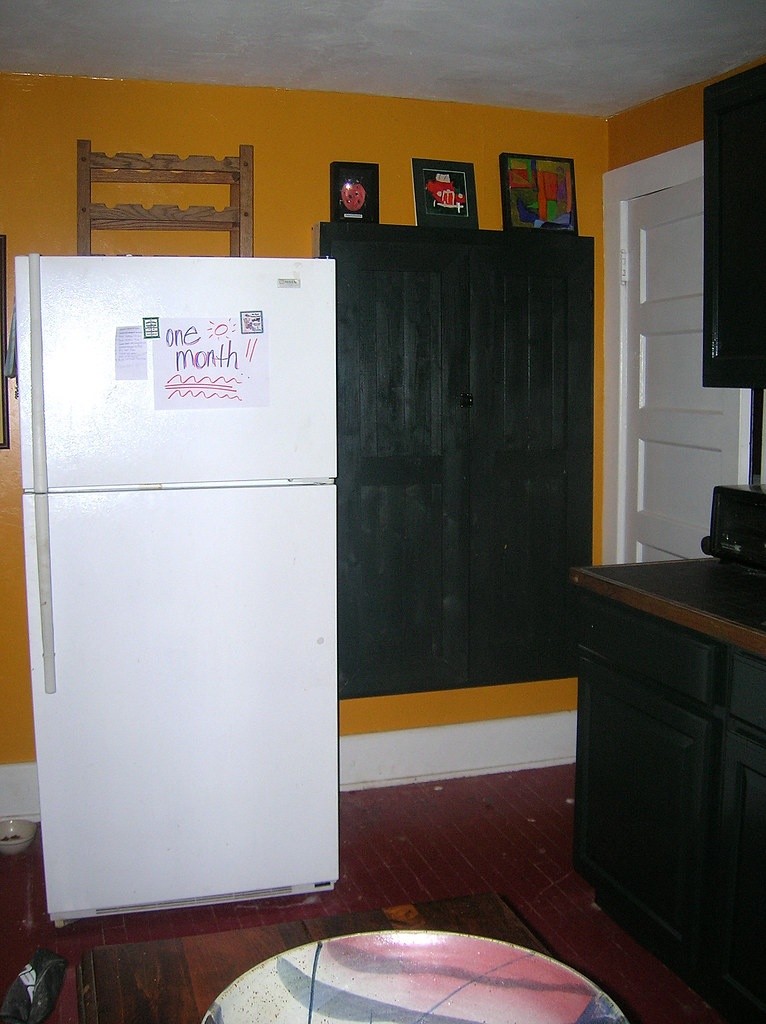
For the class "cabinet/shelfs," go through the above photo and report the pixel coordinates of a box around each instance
[320,222,595,699]
[569,557,766,1024]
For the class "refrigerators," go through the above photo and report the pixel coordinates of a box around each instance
[15,254,342,922]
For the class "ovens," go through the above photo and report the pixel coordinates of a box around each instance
[708,483,766,570]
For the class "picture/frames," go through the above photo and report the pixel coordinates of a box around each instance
[499,153,578,235]
[330,162,379,223]
[411,158,479,229]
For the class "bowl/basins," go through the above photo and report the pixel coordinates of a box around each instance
[0,818,37,854]
